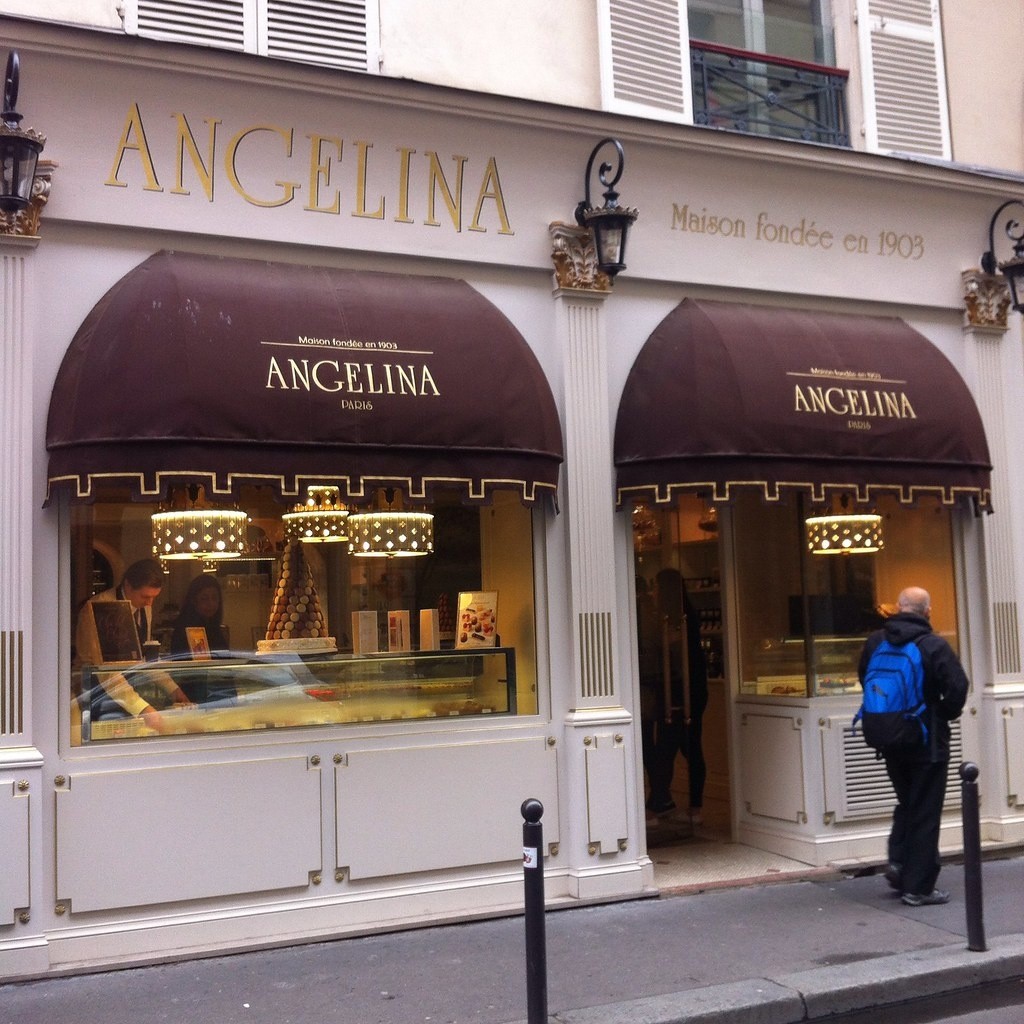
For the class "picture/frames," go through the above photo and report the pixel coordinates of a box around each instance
[186,627,212,661]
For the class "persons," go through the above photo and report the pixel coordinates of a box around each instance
[851,586,969,906]
[71,559,191,734]
[164,574,236,706]
[635,569,708,829]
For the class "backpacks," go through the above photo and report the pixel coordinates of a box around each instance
[851,631,934,761]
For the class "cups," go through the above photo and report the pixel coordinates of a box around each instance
[143,644,162,662]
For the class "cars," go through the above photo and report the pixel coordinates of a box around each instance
[67,647,341,741]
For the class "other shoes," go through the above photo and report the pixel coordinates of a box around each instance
[646,817,661,829]
[672,811,703,824]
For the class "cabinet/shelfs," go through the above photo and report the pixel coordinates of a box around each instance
[632,493,724,681]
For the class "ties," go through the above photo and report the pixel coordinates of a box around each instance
[134,610,140,638]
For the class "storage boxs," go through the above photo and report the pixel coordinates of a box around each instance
[387,610,410,653]
[420,610,441,651]
[351,610,379,654]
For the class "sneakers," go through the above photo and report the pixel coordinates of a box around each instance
[902,887,950,906]
[885,864,904,890]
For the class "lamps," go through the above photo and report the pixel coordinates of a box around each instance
[152,482,250,559]
[549,137,639,300]
[282,486,350,543]
[962,198,1024,335]
[159,559,220,574]
[800,498,884,556]
[347,488,436,559]
[0,50,59,248]
